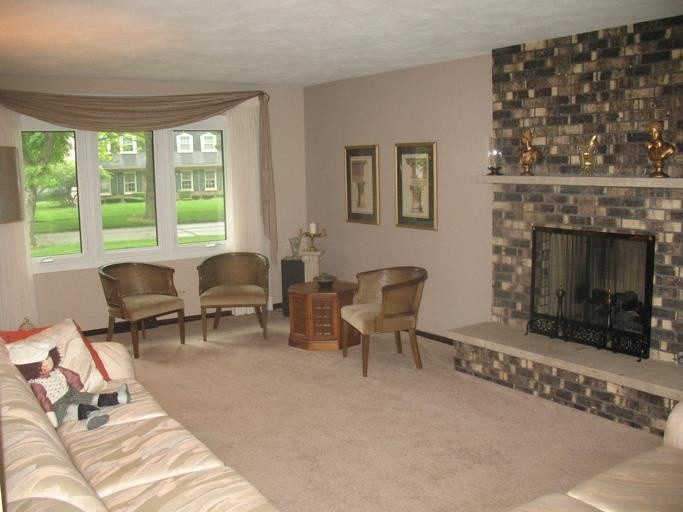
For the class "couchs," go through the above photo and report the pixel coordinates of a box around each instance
[2,339,277,510]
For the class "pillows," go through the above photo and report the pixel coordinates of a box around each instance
[0,318,110,416]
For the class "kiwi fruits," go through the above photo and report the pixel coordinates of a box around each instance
[317,273,330,280]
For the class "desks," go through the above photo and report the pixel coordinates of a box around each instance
[287,280,362,352]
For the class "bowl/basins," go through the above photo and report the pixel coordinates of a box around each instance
[313,276,337,288]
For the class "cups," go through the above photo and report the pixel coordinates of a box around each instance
[288,238,300,257]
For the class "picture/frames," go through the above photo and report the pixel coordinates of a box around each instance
[345,144,381,227]
[394,141,436,230]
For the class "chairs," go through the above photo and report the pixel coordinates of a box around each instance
[339,265,428,377]
[96,262,189,358]
[196,252,271,341]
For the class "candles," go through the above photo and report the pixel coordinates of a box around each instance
[309,222,317,232]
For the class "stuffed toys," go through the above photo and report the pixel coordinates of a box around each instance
[8,341,130,431]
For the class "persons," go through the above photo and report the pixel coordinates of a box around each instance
[646,121,676,163]
[515,128,542,166]
